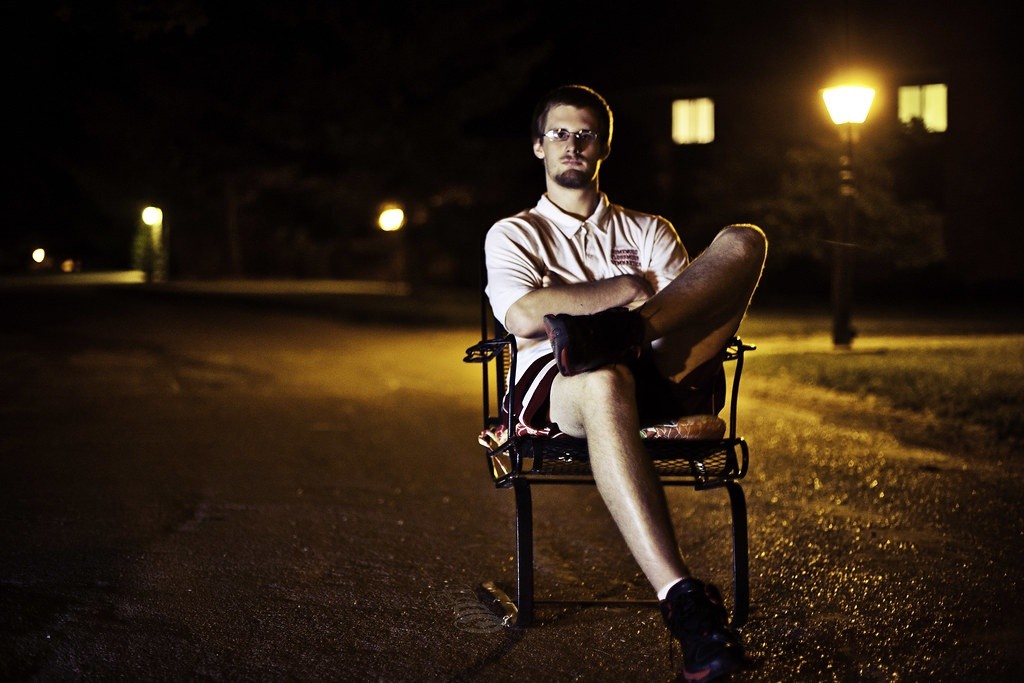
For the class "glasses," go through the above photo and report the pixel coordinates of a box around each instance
[541,128,598,144]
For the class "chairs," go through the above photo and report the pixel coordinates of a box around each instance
[463,264,756,628]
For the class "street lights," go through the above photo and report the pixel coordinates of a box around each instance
[818,66,877,346]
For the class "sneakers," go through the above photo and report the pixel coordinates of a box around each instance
[543,304,636,375]
[664,581,748,683]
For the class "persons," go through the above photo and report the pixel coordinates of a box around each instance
[484,84,771,683]
